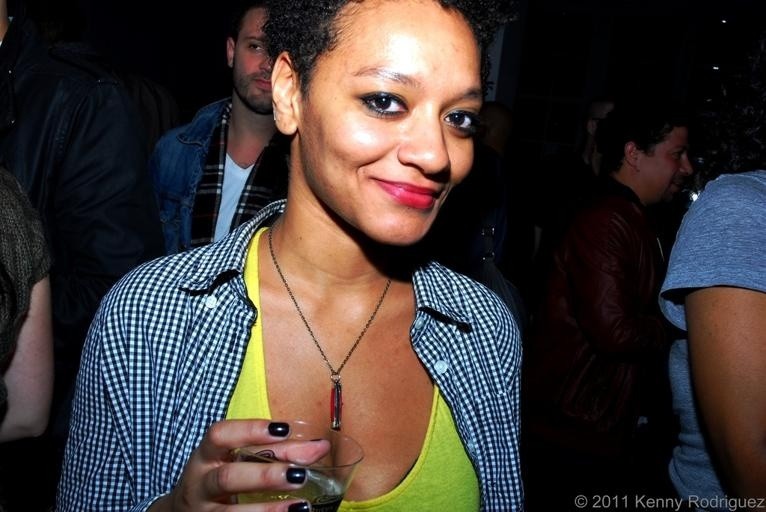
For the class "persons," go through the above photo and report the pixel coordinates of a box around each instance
[0,0,765,510]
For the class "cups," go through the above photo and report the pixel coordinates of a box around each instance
[230,422,365,510]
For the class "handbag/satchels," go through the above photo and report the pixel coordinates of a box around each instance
[477,222,525,334]
